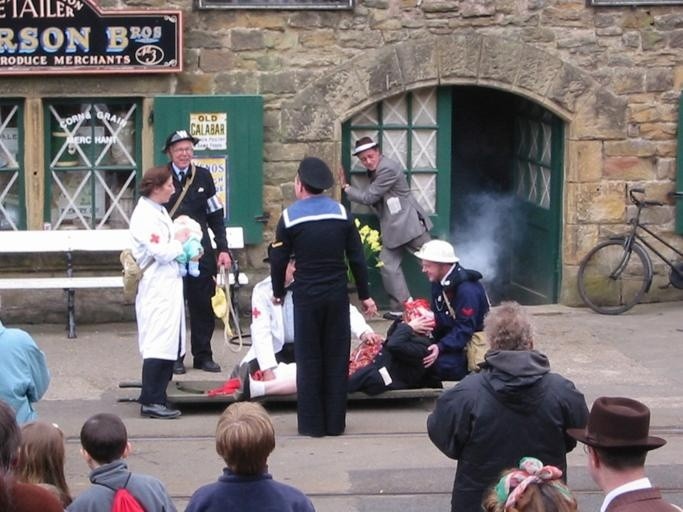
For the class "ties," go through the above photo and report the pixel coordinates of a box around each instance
[179,172,184,183]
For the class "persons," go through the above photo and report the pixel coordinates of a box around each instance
[157,130,231,374]
[414,239,490,381]
[427,300,589,511]
[483,457,577,511]
[229,300,437,402]
[271,158,377,436]
[235,241,380,382]
[65,413,177,512]
[171,215,203,278]
[126,167,205,420]
[1,321,72,510]
[184,401,315,512]
[339,136,434,312]
[566,396,682,511]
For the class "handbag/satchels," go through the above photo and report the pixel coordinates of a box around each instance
[467,332,491,371]
[119,248,143,296]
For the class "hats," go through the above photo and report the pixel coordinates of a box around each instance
[352,136,380,156]
[413,240,459,264]
[567,397,667,450]
[161,129,199,152]
[298,157,333,190]
[263,242,296,263]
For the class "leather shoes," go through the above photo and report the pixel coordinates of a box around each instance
[140,404,182,418]
[173,361,185,374]
[193,359,220,372]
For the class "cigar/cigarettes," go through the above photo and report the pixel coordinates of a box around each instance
[374,311,381,317]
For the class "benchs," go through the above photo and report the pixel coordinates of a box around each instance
[1,226,249,338]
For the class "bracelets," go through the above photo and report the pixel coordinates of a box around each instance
[342,184,349,191]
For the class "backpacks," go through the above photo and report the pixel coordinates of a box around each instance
[92,472,146,512]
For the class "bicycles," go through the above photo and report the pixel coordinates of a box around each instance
[577,185,683,314]
